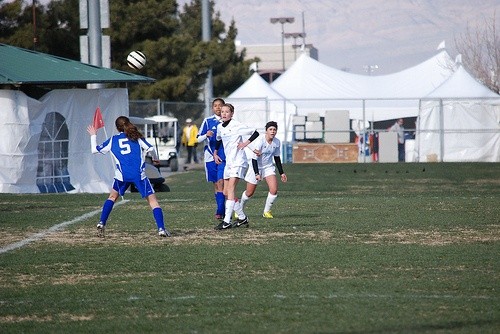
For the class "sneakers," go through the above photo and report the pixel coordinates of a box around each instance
[97,224,105,237]
[263,211,273,218]
[232,218,249,228]
[215,214,221,219]
[158,227,170,236]
[214,221,232,230]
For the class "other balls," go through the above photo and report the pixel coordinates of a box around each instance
[127,50,146,70]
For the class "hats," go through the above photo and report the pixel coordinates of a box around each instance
[186,118,192,123]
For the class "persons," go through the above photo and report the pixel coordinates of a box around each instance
[181,118,199,164]
[390,118,405,162]
[87,116,171,237]
[214,103,260,230]
[196,98,232,220]
[235,121,287,218]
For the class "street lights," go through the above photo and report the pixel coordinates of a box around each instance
[284,32,306,61]
[271,17,295,71]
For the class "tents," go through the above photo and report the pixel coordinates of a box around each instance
[0,42,158,193]
[270,51,458,163]
[224,71,297,164]
[414,66,500,162]
